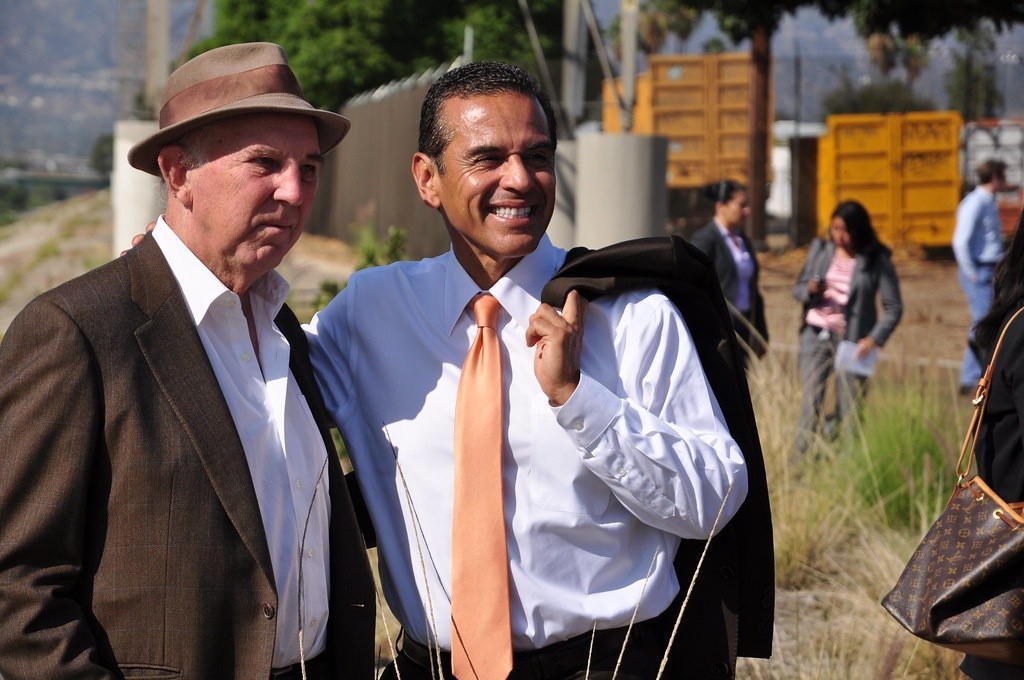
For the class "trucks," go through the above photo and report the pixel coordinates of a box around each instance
[959,118,1024,244]
[814,112,962,253]
[574,52,775,197]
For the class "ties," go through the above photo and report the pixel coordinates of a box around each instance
[449,291,512,680]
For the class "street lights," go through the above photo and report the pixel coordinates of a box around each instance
[996,49,1020,118]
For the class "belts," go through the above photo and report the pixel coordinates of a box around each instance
[402,618,658,676]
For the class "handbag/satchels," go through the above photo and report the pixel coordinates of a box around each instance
[882,305,1024,666]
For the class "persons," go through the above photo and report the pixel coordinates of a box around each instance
[689,178,771,389]
[789,199,906,477]
[961,209,1024,680]
[111,60,748,680]
[952,157,1011,397]
[0,43,408,680]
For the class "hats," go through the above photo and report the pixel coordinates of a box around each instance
[127,42,351,176]
